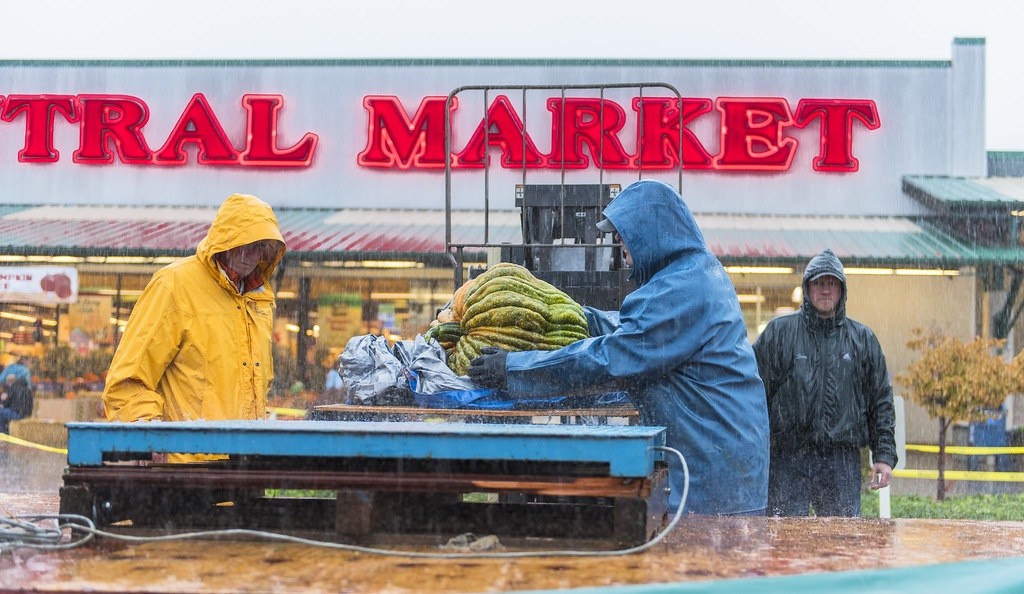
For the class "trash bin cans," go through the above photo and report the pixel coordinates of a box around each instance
[967,405,1008,472]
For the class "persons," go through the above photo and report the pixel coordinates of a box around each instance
[103,192,285,463]
[753,248,899,515]
[0,356,33,432]
[468,180,768,518]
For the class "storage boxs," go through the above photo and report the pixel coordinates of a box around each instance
[8,397,104,465]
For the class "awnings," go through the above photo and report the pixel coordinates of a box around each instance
[906,176,1024,214]
[0,202,1024,268]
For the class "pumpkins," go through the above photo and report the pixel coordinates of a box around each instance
[424,262,588,376]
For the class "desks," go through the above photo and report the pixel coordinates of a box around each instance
[314,401,639,425]
